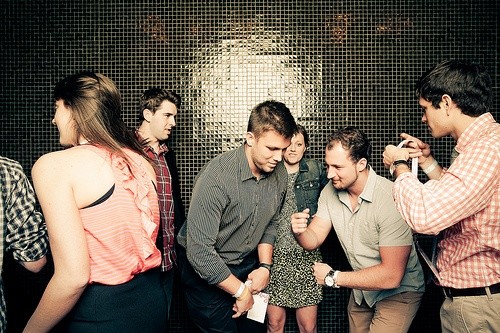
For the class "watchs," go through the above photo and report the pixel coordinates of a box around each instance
[390,160,408,176]
[324,270,335,288]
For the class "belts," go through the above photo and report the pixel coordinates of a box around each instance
[444,283,500,297]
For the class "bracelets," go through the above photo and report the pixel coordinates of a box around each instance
[259,263,273,274]
[232,283,245,298]
[334,270,341,289]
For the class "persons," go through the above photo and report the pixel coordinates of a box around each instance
[133,86,186,333]
[261,126,331,333]
[291,126,426,333]
[382,57,500,333]
[0,157,49,333]
[177,101,299,333]
[24,70,168,333]
